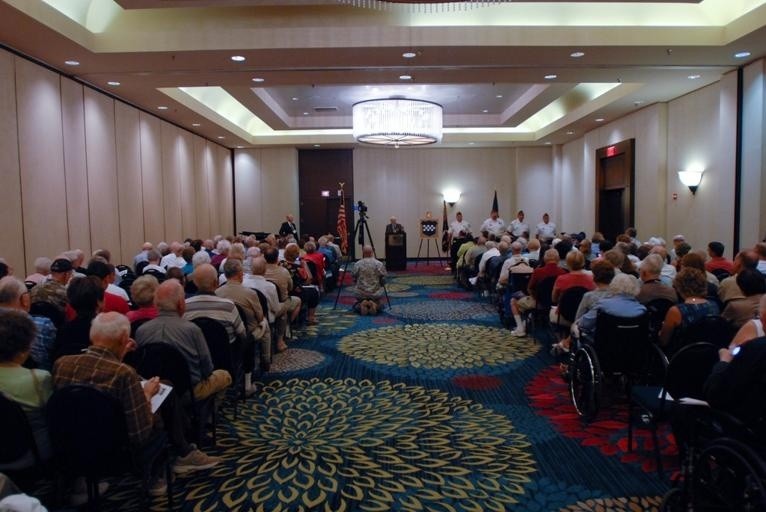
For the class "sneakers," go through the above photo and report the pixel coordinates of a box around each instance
[510,328,526,337]
[246,383,256,396]
[173,444,220,472]
[149,464,176,497]
[70,477,110,507]
[552,339,570,353]
[469,277,477,285]
[276,339,289,351]
[360,300,377,315]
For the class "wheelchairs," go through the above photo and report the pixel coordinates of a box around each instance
[671,401,764,512]
[566,309,670,428]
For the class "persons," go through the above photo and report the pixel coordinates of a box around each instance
[135,279,212,387]
[700,336,766,396]
[756,243,765,272]
[279,218,299,241]
[52,311,222,473]
[27,231,340,350]
[180,263,258,397]
[722,268,765,325]
[449,210,756,374]
[349,244,389,316]
[385,217,405,232]
[1,275,60,370]
[55,277,104,356]
[214,259,270,372]
[658,268,720,384]
[2,261,28,294]
[1,310,52,472]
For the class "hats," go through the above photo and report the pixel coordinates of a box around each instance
[50,258,75,272]
[643,237,665,245]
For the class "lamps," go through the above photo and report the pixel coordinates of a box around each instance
[676,168,705,197]
[350,95,446,151]
[439,187,464,208]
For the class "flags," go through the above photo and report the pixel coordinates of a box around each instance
[337,189,350,255]
[441,202,449,253]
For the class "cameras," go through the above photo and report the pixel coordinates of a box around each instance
[358,199,368,211]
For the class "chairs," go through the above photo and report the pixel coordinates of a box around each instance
[450,231,765,477]
[0,239,343,512]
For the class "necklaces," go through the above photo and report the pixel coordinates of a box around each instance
[692,298,695,301]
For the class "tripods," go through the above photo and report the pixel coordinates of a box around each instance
[333,211,392,309]
